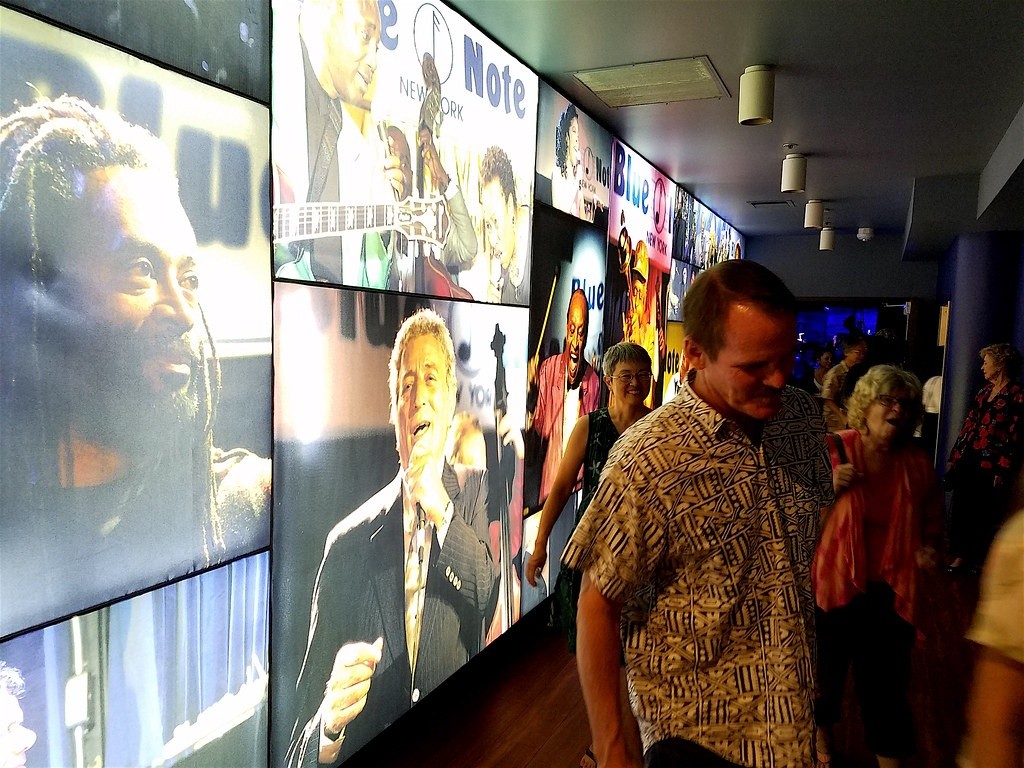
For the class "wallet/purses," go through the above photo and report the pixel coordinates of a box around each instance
[944,468,954,492]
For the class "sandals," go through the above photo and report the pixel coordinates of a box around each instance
[581,748,597,767]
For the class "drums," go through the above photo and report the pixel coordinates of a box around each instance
[522,492,578,617]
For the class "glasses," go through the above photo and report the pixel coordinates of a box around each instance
[853,349,867,356]
[879,393,898,407]
[610,371,652,383]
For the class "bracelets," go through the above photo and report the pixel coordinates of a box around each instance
[323,722,346,742]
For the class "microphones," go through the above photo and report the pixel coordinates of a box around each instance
[415,502,426,564]
[576,151,582,190]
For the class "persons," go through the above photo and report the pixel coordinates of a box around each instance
[809,349,833,395]
[272,0,530,306]
[526,102,745,603]
[0,95,271,635]
[811,364,925,768]
[0,660,37,768]
[946,344,1024,574]
[283,305,526,768]
[559,260,836,768]
[956,508,1024,768]
[823,335,868,432]
[526,342,654,768]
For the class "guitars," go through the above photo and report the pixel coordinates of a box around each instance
[274,193,453,250]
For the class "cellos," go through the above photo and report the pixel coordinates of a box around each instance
[399,51,473,298]
[484,322,523,645]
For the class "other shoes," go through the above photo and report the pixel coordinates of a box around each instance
[946,563,960,575]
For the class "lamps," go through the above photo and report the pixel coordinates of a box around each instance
[780,153,808,195]
[802,200,823,229]
[738,64,775,125]
[820,223,833,252]
